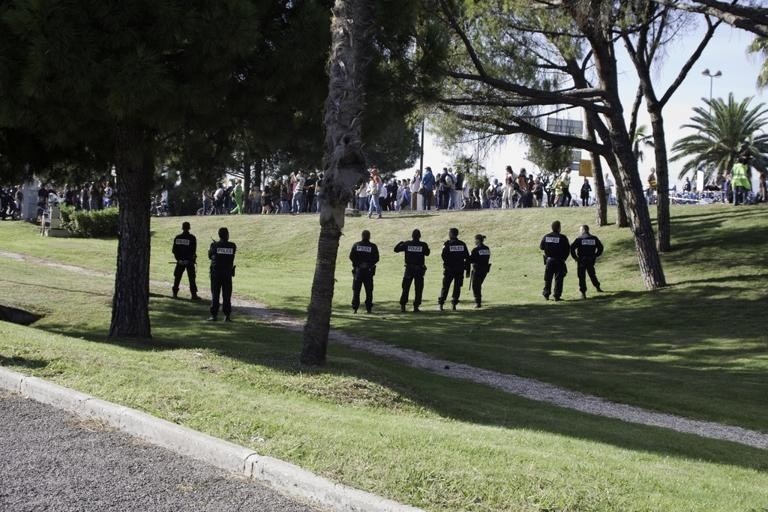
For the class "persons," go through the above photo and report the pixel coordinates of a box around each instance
[570,225,604,300]
[348,230,379,314]
[437,228,470,311]
[469,234,490,308]
[152,169,323,216]
[393,229,429,312]
[356,165,572,218]
[580,158,767,206]
[171,222,201,302]
[1,180,115,220]
[539,220,569,301]
[204,227,236,323]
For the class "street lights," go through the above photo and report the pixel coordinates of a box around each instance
[701,67,721,184]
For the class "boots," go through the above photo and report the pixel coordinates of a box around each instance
[224,316,231,322]
[191,291,201,301]
[541,286,604,302]
[173,293,179,298]
[352,302,482,314]
[206,315,218,321]
